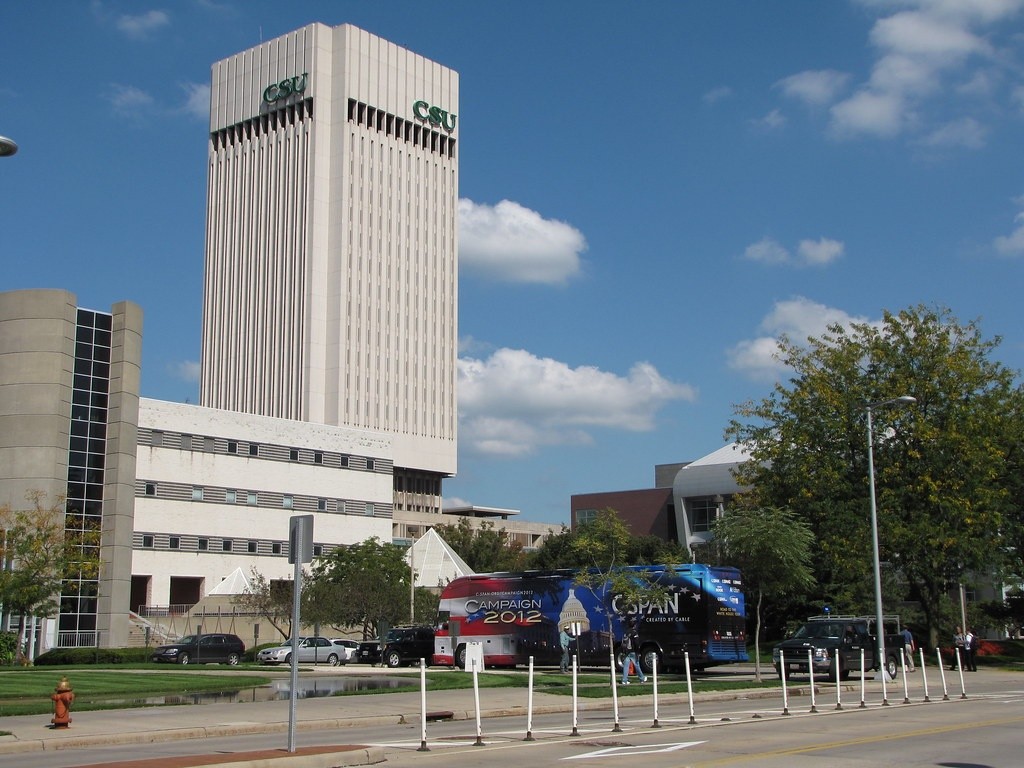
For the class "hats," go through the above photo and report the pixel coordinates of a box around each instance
[564,625,569,629]
[902,625,908,629]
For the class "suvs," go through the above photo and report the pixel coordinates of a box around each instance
[151,633,247,666]
[355,625,435,668]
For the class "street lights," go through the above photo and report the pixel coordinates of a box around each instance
[865,395,918,683]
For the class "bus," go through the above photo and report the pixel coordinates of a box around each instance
[432,563,749,676]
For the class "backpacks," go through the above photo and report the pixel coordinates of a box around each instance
[621,630,636,653]
[965,635,978,650]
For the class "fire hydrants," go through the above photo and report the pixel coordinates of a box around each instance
[49,674,75,730]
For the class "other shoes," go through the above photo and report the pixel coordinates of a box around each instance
[560,671,568,674]
[641,677,647,683]
[961,666,964,671]
[966,669,976,672]
[906,671,908,673]
[910,669,917,673]
[565,670,572,674]
[622,681,630,685]
[950,667,955,670]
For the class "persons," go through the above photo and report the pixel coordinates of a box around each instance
[959,627,978,671]
[949,626,964,671]
[559,626,577,674]
[900,625,917,672]
[846,625,856,638]
[618,635,647,684]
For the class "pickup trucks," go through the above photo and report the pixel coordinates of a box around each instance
[773,614,907,682]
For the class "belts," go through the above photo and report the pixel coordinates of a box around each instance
[905,643,910,644]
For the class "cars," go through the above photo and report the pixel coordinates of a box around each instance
[328,638,360,663]
[256,635,347,667]
